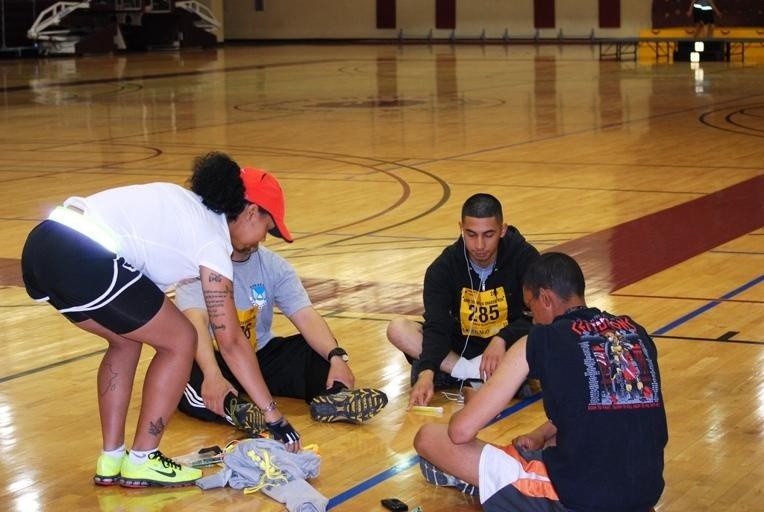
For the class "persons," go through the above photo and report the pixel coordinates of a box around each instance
[410,252,670,512]
[21,149,304,490]
[173,242,388,454]
[386,195,542,414]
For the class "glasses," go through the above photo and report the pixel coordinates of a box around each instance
[521,287,551,317]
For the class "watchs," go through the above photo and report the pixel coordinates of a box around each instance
[325,346,351,362]
[258,399,280,414]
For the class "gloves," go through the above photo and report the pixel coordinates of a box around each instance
[265,416,300,444]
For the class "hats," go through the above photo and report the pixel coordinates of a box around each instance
[240,166,293,243]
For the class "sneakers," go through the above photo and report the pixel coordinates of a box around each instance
[119,450,203,488]
[309,387,388,423]
[419,457,479,497]
[92,448,129,486]
[225,398,272,439]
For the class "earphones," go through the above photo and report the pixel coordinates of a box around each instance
[461,227,464,237]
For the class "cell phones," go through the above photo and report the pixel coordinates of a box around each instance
[463,378,484,390]
[381,499,408,512]
